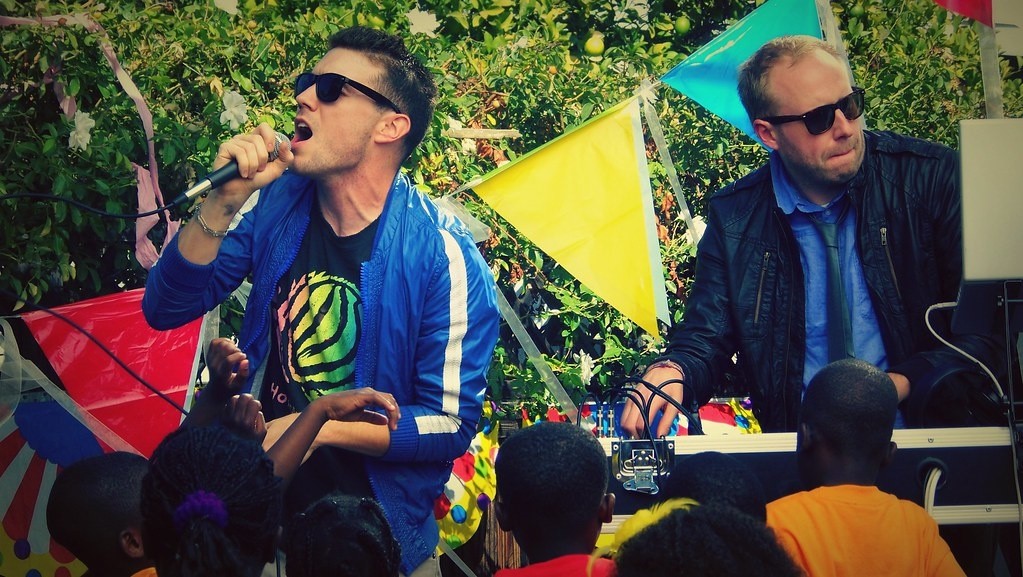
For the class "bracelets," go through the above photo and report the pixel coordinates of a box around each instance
[194,204,226,237]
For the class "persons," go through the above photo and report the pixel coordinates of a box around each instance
[142,28,500,577]
[46,337,401,577]
[621,34,1017,577]
[495,421,806,577]
[765,359,966,577]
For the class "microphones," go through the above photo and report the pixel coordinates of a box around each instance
[172,133,291,206]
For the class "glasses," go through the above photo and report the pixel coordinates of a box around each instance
[761,86,865,135]
[295,73,402,116]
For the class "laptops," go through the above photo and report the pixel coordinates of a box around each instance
[950,117,1023,335]
